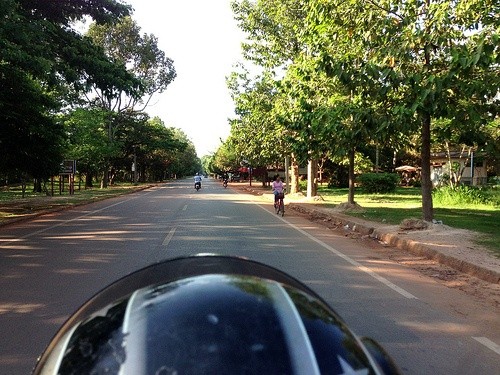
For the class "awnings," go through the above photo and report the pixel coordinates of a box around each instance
[237,168,248,172]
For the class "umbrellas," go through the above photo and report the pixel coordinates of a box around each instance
[395,166,416,173]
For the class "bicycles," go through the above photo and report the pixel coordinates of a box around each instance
[273,188,287,216]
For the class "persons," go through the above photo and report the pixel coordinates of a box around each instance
[272,174,284,208]
[194,173,201,188]
[223,174,229,181]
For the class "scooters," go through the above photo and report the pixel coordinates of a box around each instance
[194,179,202,191]
[223,178,228,189]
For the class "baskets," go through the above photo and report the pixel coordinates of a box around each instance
[277,192,284,198]
